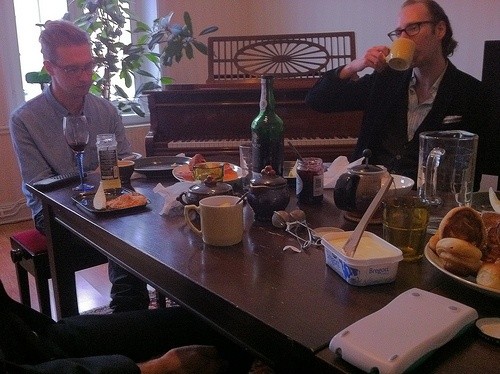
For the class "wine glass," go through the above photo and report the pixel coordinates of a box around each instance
[63,116,95,190]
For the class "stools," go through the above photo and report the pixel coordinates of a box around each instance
[10,228,108,319]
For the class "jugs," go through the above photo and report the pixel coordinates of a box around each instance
[334,149,397,224]
[244,165,290,221]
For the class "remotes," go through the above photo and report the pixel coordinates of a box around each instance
[33,171,87,189]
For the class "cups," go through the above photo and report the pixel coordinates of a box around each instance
[117,160,135,193]
[381,195,431,263]
[184,196,243,247]
[239,145,253,192]
[97,134,122,195]
[417,130,479,236]
[193,163,225,182]
[379,37,416,71]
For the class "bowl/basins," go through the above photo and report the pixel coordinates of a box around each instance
[171,162,243,186]
[389,173,415,199]
[133,156,193,177]
[321,231,402,287]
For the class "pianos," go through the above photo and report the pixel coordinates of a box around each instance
[142,76,360,154]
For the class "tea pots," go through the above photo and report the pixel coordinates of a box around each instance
[176,176,240,217]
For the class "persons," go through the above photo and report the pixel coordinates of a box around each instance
[9,19,151,311]
[1,279,270,374]
[303,0,493,193]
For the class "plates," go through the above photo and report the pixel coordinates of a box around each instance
[71,187,152,213]
[425,236,500,299]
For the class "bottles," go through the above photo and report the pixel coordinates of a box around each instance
[296,158,324,204]
[251,74,284,177]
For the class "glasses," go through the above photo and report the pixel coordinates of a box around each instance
[49,60,100,78]
[388,21,434,42]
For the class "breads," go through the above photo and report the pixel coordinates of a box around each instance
[428,206,500,290]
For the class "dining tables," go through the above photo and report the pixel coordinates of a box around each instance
[26,155,500,374]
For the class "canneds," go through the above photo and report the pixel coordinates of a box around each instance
[95,133,122,189]
[295,157,324,204]
